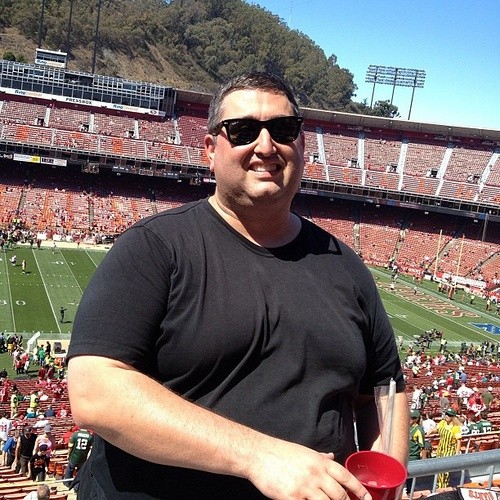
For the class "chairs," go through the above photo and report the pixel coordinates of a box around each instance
[0,362,500,500]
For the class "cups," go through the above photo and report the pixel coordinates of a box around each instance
[345,451,407,500]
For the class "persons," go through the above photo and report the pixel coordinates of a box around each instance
[0,190,500,500]
[65,72,409,500]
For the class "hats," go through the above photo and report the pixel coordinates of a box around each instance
[443,409,456,416]
[411,410,420,417]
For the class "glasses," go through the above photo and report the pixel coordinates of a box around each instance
[211,116,304,145]
